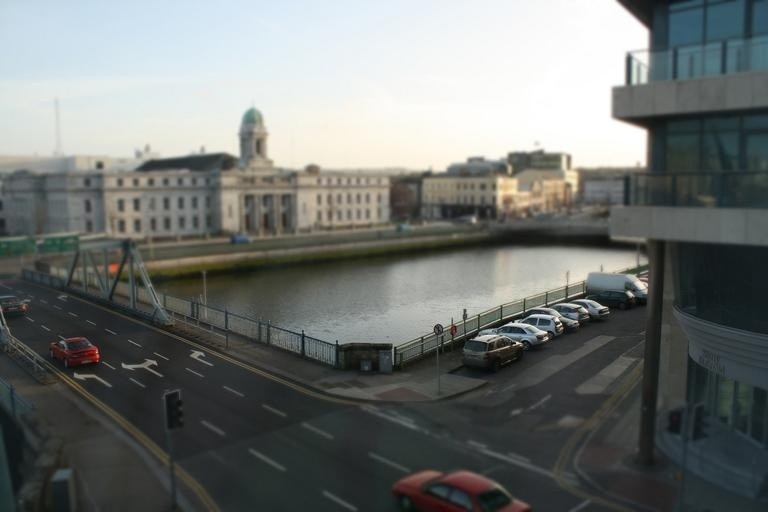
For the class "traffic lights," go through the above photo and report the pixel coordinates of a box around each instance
[667,408,682,436]
[44,234,79,254]
[695,408,710,442]
[164,389,184,431]
[0,236,36,258]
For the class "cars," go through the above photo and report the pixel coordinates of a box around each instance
[0,295,28,318]
[479,271,648,350]
[49,337,101,369]
[392,467,533,512]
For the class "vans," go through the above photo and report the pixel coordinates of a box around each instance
[231,234,249,243]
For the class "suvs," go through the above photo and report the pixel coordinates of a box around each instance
[459,331,523,374]
